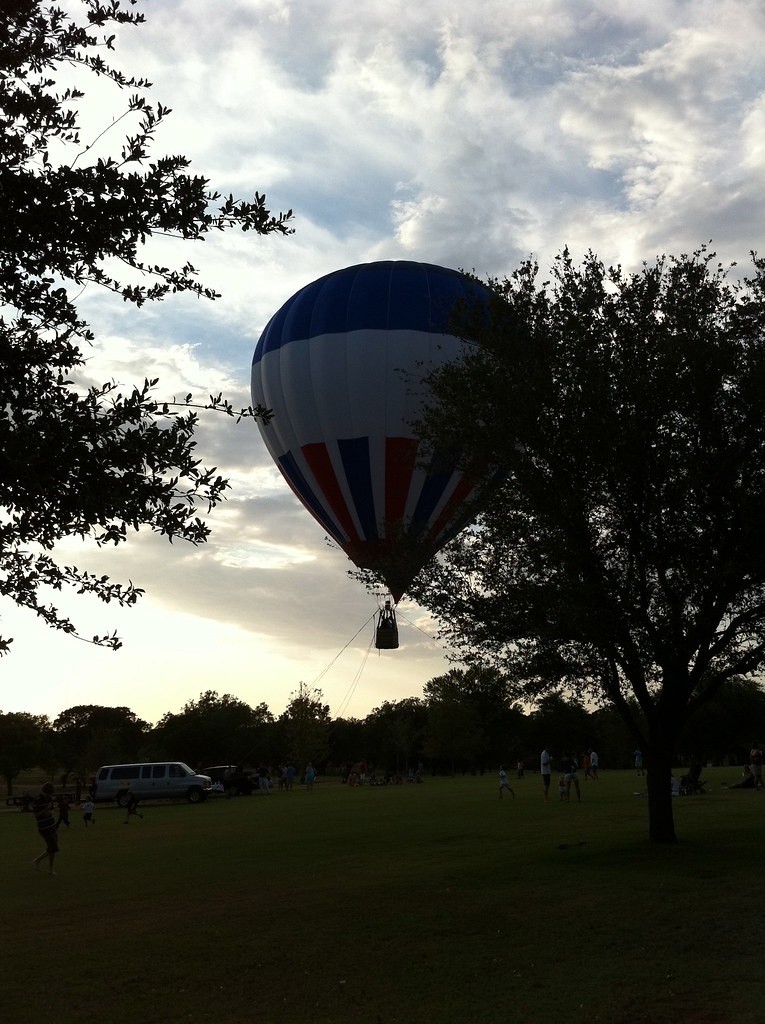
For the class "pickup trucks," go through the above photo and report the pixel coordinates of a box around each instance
[197,766,261,795]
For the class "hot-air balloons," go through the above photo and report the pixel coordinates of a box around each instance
[250,257,546,650]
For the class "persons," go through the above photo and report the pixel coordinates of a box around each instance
[255,762,295,792]
[558,751,581,802]
[123,792,144,824]
[687,755,705,794]
[583,752,595,781]
[32,783,60,875]
[300,760,320,791]
[80,793,96,827]
[498,765,515,800]
[541,745,553,801]
[588,749,598,780]
[517,759,524,778]
[55,800,71,827]
[342,758,423,786]
[380,616,391,628]
[634,745,645,777]
[727,741,763,790]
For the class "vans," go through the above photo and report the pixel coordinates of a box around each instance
[88,762,212,806]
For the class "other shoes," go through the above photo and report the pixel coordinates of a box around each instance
[267,792,271,794]
[140,813,143,819]
[641,771,645,776]
[637,774,640,776]
[123,822,129,824]
